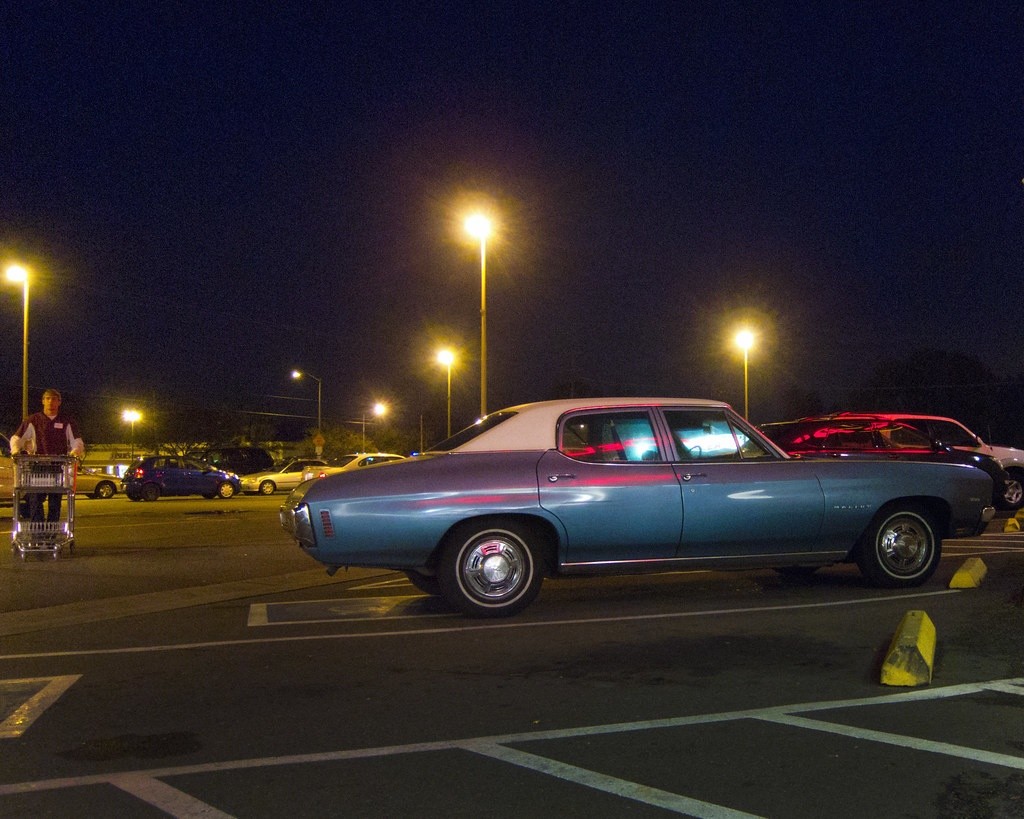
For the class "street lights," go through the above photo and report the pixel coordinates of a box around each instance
[467,211,490,421]
[728,322,765,426]
[5,261,31,425]
[293,370,322,435]
[123,407,144,465]
[361,403,386,452]
[439,346,453,438]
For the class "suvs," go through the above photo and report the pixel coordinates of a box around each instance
[184,448,275,478]
[795,411,1024,509]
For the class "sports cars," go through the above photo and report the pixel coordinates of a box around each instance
[280,398,996,621]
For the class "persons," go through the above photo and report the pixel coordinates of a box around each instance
[10,388,83,551]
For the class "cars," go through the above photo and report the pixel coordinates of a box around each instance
[737,417,1010,517]
[124,455,242,502]
[237,458,329,495]
[300,452,409,486]
[0,434,29,500]
[74,463,126,500]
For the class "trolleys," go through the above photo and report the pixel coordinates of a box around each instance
[9,453,78,561]
